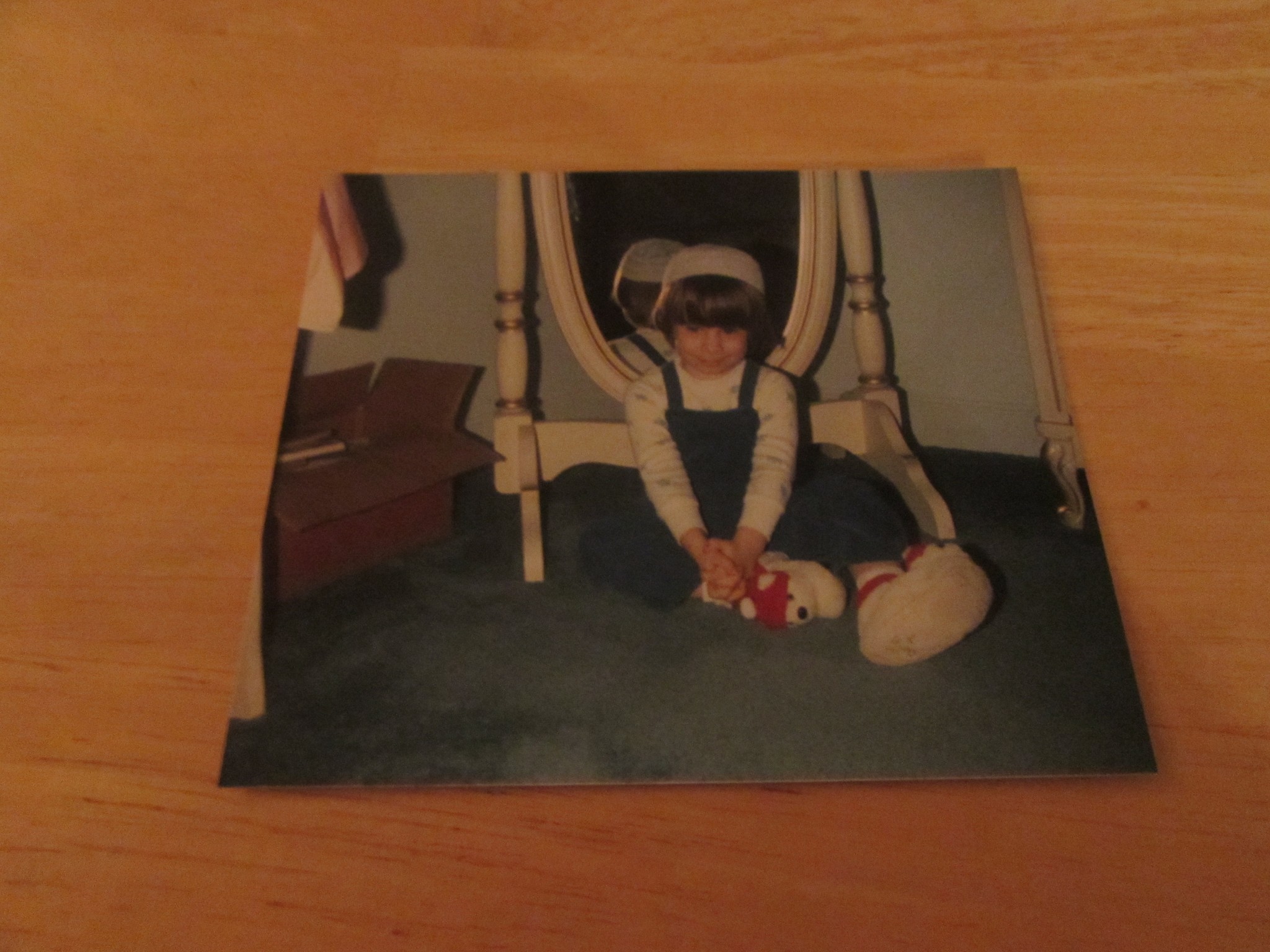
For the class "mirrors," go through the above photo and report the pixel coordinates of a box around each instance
[491,171,957,583]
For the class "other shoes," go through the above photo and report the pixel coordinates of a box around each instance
[737,551,846,626]
[853,542,992,666]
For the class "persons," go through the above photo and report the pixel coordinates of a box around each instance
[609,239,687,379]
[569,238,996,667]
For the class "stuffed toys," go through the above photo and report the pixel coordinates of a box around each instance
[701,548,845,626]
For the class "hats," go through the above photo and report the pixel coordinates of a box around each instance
[620,239,682,282]
[662,242,766,294]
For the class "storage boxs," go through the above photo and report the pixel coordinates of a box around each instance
[271,356,506,606]
